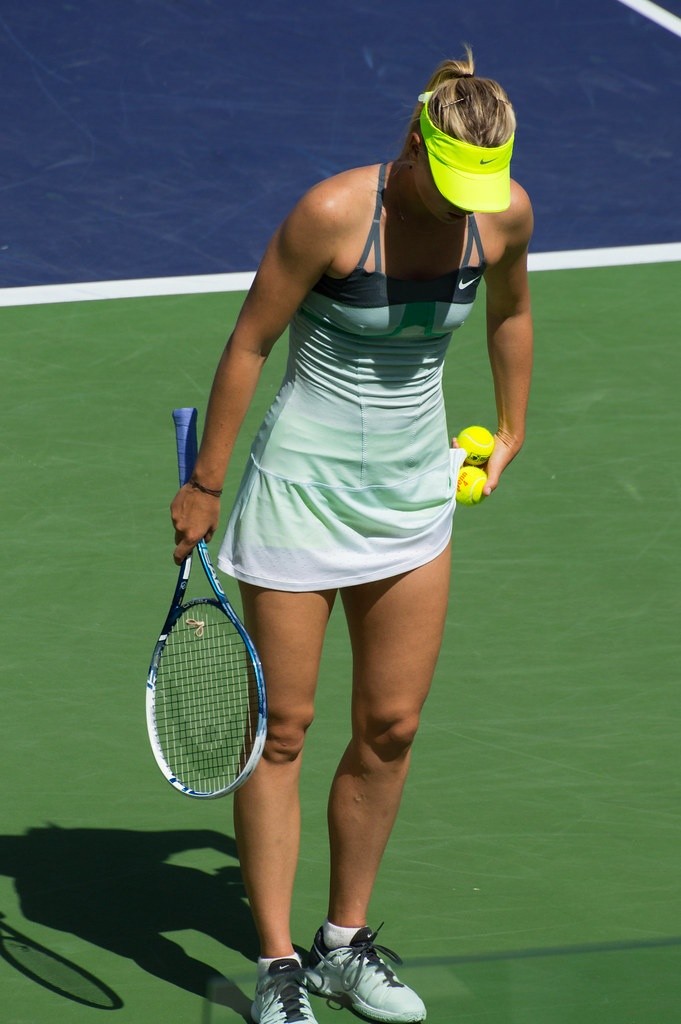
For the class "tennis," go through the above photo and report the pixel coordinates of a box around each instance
[454,466,490,506]
[457,426,493,465]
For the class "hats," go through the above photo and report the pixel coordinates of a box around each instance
[417,91,516,213]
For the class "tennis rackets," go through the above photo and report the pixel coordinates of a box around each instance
[147,407,270,803]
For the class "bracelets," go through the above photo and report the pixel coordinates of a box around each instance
[188,477,223,499]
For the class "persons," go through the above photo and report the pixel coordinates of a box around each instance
[170,41,534,1024]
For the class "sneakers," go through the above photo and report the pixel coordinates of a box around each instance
[300,922,427,1022]
[250,958,322,1024]
